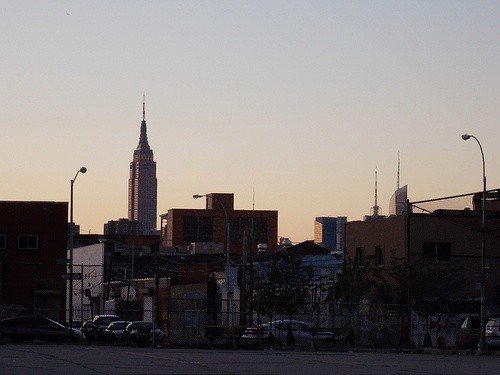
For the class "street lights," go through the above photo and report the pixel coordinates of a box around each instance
[461,134,489,357]
[193,194,231,346]
[69,167,89,346]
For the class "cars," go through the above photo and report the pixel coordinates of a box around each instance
[0,315,87,346]
[242,320,336,350]
[66,314,165,350]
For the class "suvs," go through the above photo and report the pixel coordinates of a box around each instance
[484,317,500,352]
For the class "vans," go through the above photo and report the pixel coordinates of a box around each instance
[457,315,491,352]
